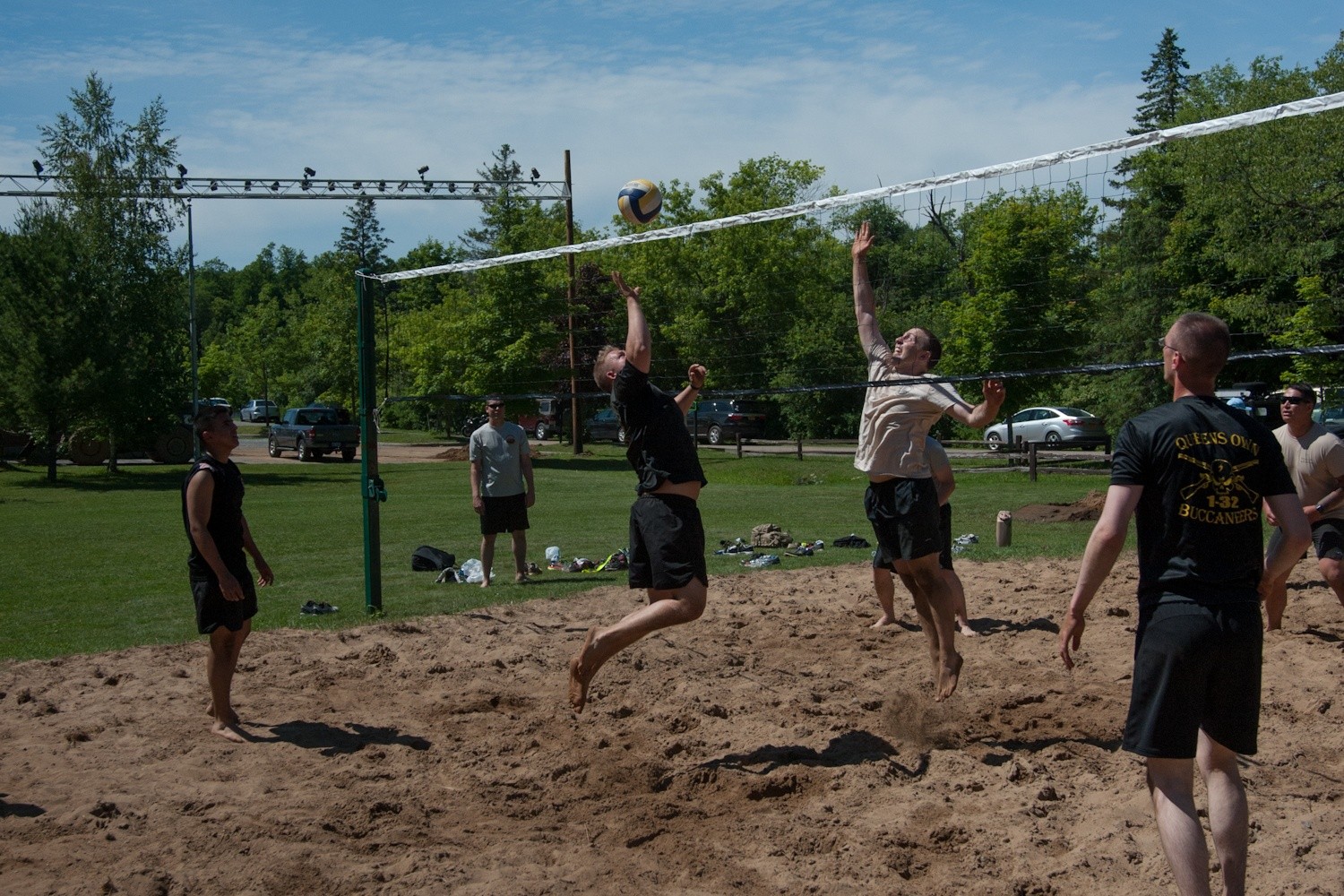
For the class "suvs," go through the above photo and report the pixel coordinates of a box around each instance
[685,399,778,447]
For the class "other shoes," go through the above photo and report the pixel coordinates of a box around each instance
[952,532,978,545]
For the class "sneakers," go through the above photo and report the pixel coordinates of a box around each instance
[547,546,630,573]
[739,552,780,569]
[524,562,542,576]
[713,537,754,556]
[300,600,339,616]
[782,539,825,557]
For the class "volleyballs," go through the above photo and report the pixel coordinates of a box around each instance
[616,178,662,225]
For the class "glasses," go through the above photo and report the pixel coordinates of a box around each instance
[1278,395,1308,404]
[487,403,504,409]
[1158,337,1186,364]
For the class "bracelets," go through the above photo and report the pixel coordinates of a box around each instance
[689,382,706,390]
[1315,502,1330,515]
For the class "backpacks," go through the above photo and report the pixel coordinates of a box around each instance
[411,545,456,571]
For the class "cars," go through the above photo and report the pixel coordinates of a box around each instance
[517,389,634,445]
[983,406,1106,453]
[200,398,362,460]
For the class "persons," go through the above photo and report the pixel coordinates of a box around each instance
[1054,311,1316,896]
[564,268,711,715]
[177,404,277,744]
[866,434,983,639]
[849,218,1009,704]
[468,393,536,589]
[1261,380,1344,632]
